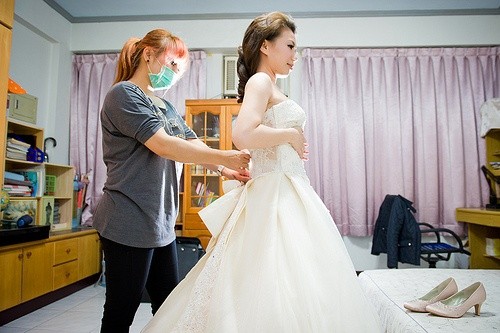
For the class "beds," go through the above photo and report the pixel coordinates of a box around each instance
[358,269,500,333]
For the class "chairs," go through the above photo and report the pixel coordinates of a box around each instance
[382,194,472,268]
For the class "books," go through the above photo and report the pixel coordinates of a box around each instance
[3,171,34,197]
[191,163,220,207]
[52,198,61,225]
[6,138,31,161]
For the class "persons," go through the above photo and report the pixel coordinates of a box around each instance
[227,11,333,333]
[94,28,251,333]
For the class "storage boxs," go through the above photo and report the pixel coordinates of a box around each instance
[46,175,56,195]
[8,92,38,125]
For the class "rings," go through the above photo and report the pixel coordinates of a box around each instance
[240,167,243,169]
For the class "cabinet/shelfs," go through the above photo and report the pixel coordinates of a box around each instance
[485,99,500,208]
[183,99,244,248]
[0,117,101,312]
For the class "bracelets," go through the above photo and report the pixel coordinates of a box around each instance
[216,165,225,177]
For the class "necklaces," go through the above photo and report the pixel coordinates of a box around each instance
[272,80,288,97]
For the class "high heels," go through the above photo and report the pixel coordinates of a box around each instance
[426,282,486,318]
[403,277,458,312]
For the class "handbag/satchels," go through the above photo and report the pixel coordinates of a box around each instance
[140,238,199,304]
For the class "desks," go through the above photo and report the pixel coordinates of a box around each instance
[455,208,500,269]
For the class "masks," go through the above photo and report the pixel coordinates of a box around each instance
[149,65,180,97]
